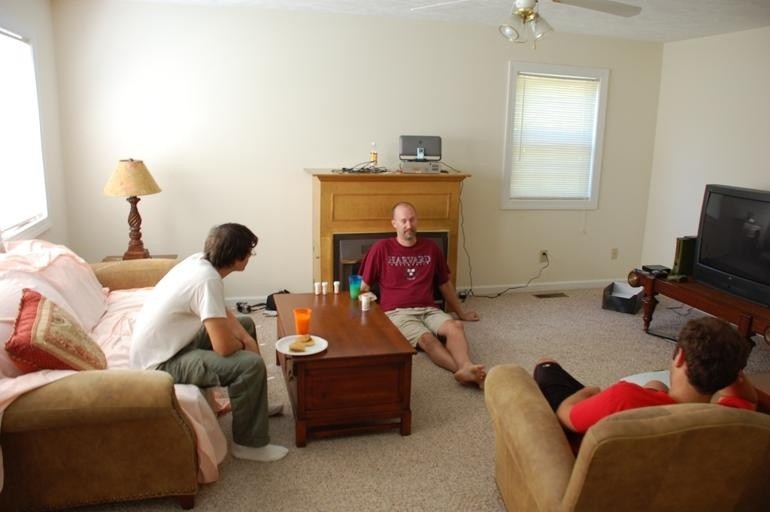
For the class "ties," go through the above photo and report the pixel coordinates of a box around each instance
[690,184,770,309]
[398,135,442,160]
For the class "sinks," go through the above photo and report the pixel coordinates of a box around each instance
[0,237,199,512]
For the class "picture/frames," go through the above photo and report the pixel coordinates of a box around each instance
[628,267,770,347]
[305,166,473,310]
[102,254,177,260]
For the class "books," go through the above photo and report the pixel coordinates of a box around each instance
[602,281,645,316]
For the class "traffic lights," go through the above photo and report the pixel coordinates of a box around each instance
[4,289,107,374]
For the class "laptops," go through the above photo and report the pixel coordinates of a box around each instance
[289,342,306,354]
[295,333,316,346]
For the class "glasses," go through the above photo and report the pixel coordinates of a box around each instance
[498,0,554,50]
[103,158,161,260]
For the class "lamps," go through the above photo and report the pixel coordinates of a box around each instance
[484,362,770,511]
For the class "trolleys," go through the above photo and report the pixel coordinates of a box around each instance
[410,0,643,32]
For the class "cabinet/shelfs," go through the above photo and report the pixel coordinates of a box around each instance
[362,294,370,310]
[334,280,341,294]
[370,141,378,166]
[321,281,329,296]
[314,282,322,295]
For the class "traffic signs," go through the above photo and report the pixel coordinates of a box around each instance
[537,249,550,266]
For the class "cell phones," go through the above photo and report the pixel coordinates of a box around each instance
[236,302,252,314]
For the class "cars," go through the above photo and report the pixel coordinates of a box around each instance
[348,275,362,299]
[292,307,312,335]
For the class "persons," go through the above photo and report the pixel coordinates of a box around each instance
[123,221,290,464]
[532,313,760,457]
[359,201,490,390]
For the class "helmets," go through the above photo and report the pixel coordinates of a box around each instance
[274,334,329,356]
